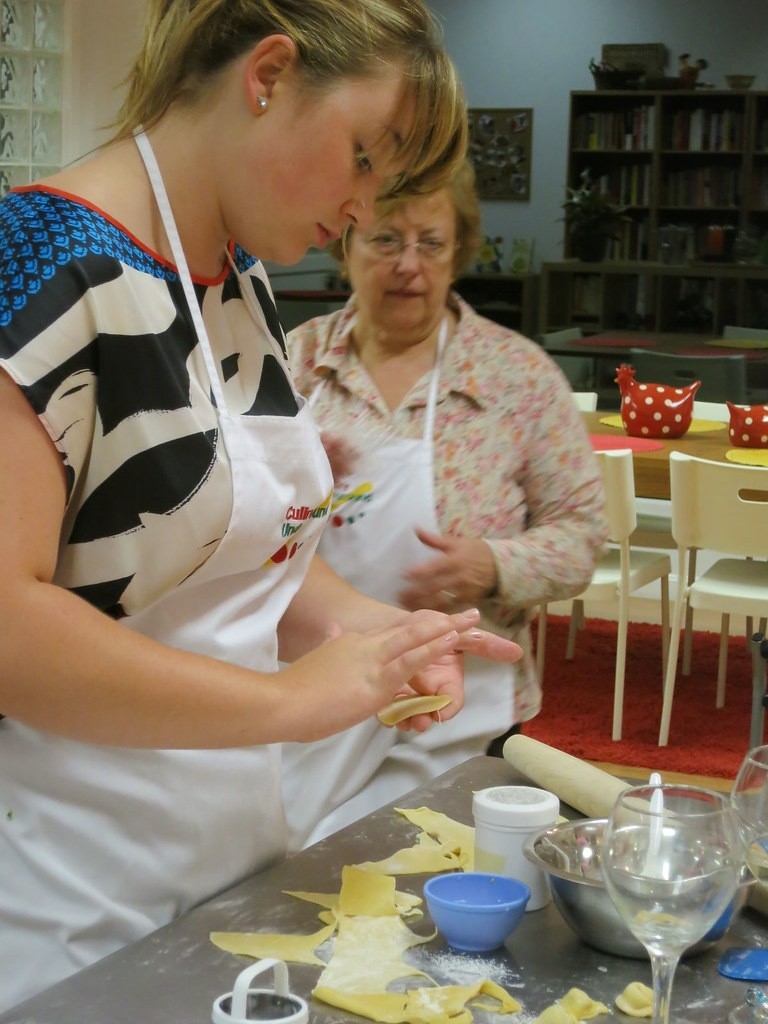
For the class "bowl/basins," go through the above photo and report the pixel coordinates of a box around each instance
[521,816,753,959]
[723,73,757,88]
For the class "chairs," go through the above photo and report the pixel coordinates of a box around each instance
[572,392,599,412]
[631,346,753,405]
[533,448,674,745]
[724,325,768,404]
[543,325,609,390]
[656,451,768,747]
[564,400,755,677]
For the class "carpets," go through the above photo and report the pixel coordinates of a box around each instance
[519,613,768,785]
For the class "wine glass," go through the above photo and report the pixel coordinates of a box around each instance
[727,745,768,1024]
[602,783,744,1024]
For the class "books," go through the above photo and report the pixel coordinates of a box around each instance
[578,105,768,260]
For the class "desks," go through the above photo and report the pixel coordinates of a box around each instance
[274,289,351,335]
[560,330,768,366]
[0,754,768,1024]
[582,411,768,502]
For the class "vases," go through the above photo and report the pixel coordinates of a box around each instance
[678,70,698,91]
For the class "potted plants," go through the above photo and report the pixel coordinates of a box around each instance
[588,55,646,91]
[554,190,636,265]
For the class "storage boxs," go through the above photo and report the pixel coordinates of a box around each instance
[599,42,671,71]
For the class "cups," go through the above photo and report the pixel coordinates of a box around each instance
[424,872,532,950]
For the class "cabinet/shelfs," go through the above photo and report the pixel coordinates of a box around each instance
[0,0,67,198]
[563,90,768,264]
[452,271,540,338]
[539,261,768,348]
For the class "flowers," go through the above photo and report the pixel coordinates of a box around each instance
[678,52,711,77]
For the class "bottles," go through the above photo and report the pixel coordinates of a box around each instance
[471,784,561,911]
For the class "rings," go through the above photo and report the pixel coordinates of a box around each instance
[440,589,456,602]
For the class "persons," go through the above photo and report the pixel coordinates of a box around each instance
[279,158,607,855]
[0,0,526,928]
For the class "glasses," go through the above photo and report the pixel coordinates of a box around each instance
[357,226,457,265]
